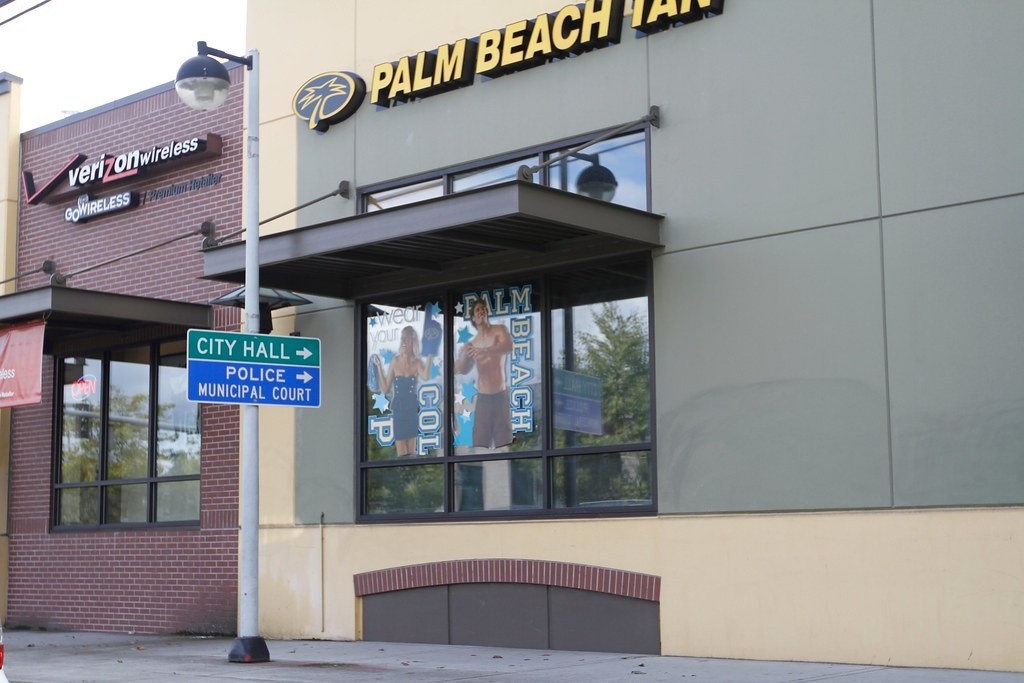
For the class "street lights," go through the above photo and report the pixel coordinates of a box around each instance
[177,39,267,662]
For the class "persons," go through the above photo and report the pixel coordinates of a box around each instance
[374,302,442,456]
[454,299,514,452]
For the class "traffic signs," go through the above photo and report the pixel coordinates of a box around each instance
[187,330,321,410]
